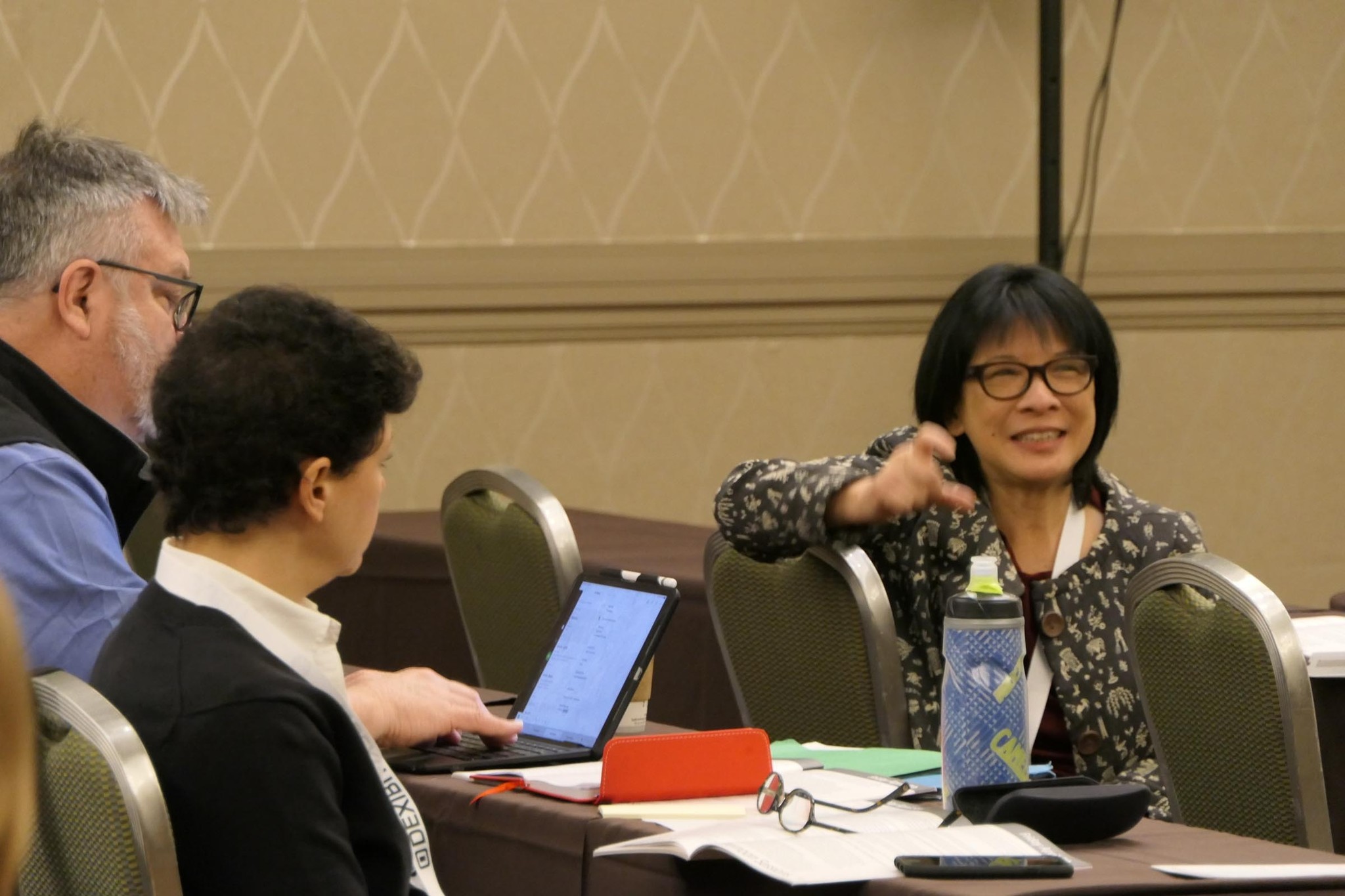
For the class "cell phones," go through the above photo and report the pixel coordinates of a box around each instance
[894,855,1075,878]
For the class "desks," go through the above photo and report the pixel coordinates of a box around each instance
[320,499,1340,736]
[368,674,1345,896]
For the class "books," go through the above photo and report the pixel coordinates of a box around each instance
[449,759,602,802]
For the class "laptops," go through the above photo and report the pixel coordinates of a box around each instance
[377,567,681,775]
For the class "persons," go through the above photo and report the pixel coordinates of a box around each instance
[87,283,445,896]
[0,121,524,751]
[713,262,1210,823]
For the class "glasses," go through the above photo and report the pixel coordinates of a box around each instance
[51,258,205,332]
[758,771,910,835]
[965,351,1099,401]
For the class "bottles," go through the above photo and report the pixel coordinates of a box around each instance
[940,557,1029,813]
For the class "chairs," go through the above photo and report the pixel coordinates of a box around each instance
[437,465,584,696]
[705,526,913,754]
[15,667,191,896]
[1123,553,1335,852]
